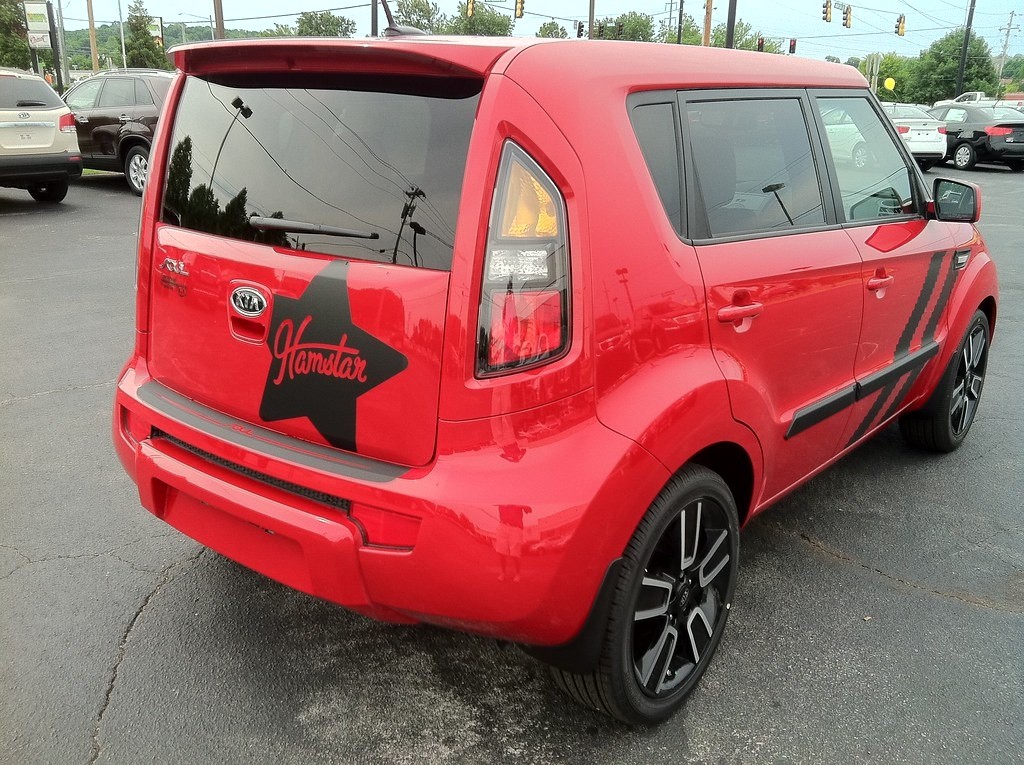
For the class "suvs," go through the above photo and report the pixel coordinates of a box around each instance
[60,68,174,196]
[938,190,962,203]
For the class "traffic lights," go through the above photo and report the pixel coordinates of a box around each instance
[822,1,831,23]
[598,25,603,36]
[618,23,623,35]
[895,16,906,36]
[789,39,796,54]
[577,22,584,38]
[758,38,764,52]
[843,6,852,29]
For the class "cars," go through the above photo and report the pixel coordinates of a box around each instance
[0,66,82,204]
[110,32,998,730]
[928,104,1024,171]
[818,101,947,172]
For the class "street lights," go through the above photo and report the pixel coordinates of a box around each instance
[761,183,797,226]
[198,94,252,230]
[178,13,215,41]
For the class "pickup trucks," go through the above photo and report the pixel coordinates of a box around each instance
[934,92,1024,113]
